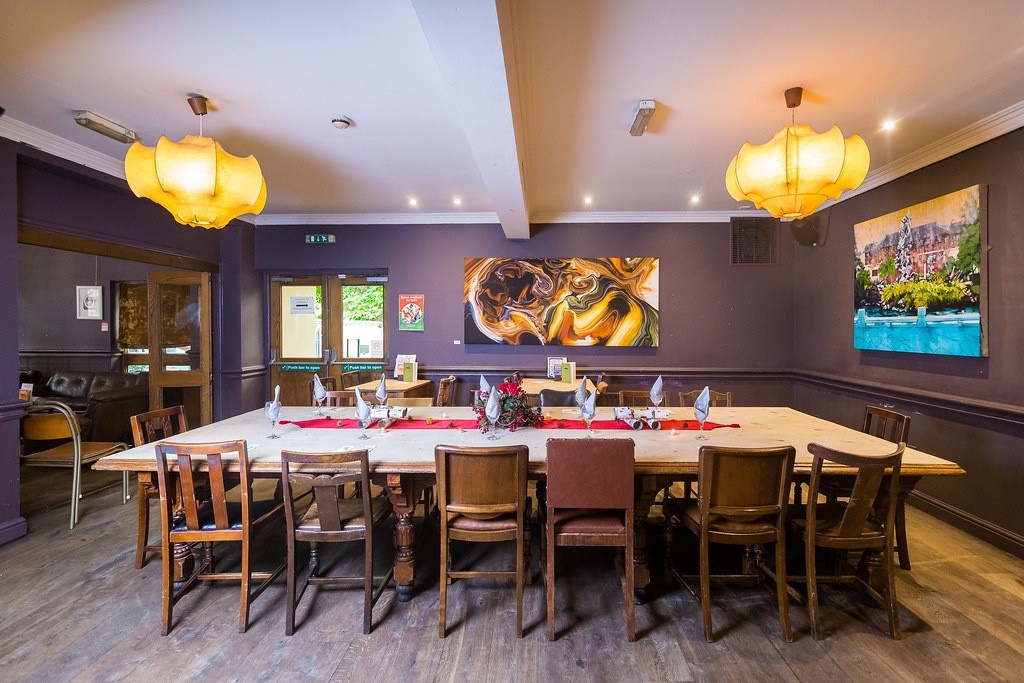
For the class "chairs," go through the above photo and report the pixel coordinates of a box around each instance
[386,376,457,406]
[154,440,287,637]
[21,401,128,529]
[619,390,732,407]
[435,444,531,637]
[795,405,911,508]
[788,442,907,640]
[503,371,608,407]
[664,446,797,643]
[130,404,253,569]
[278,448,395,635]
[539,439,635,641]
[310,370,361,407]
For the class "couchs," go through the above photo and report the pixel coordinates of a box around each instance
[40,369,149,443]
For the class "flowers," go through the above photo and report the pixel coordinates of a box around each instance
[471,381,545,431]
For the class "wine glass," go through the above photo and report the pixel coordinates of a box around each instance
[313,387,326,416]
[375,387,387,405]
[355,401,371,440]
[264,402,281,439]
[485,404,501,440]
[650,391,664,409]
[575,392,587,409]
[581,406,596,438]
[693,413,709,441]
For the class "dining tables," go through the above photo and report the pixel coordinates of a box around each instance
[92,406,968,591]
[345,379,431,406]
[519,377,600,406]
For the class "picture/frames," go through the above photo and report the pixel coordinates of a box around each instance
[853,184,988,357]
[75,286,103,319]
[463,257,660,348]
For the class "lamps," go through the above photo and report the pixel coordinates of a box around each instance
[125,98,267,229]
[630,100,657,137]
[725,88,871,222]
[74,111,138,142]
